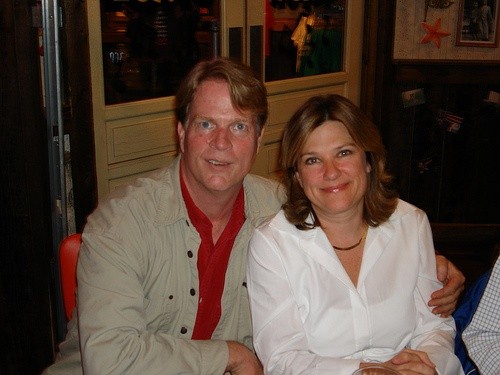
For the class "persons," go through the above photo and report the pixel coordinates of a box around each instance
[36,59,465,375]
[463,254,500,375]
[247,91,465,375]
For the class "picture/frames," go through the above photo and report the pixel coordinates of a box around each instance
[456,0,500,48]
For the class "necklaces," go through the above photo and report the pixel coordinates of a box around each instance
[334,237,363,250]
